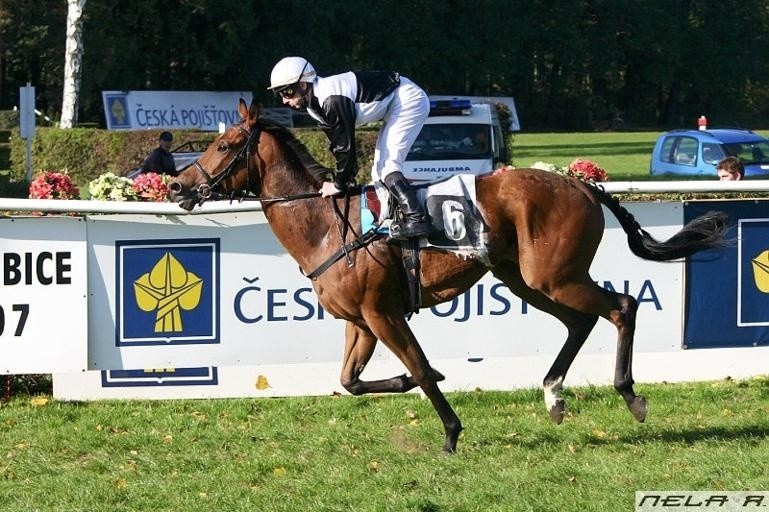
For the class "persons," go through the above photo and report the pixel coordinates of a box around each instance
[139,130,177,178]
[265,56,437,241]
[715,156,745,182]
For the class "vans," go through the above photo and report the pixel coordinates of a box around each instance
[403,100,506,185]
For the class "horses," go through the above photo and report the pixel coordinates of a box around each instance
[167,98,737,455]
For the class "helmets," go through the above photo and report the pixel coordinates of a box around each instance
[266,56,318,91]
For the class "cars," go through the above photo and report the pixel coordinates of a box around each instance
[650,129,769,177]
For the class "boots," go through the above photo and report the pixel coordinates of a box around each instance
[387,177,436,240]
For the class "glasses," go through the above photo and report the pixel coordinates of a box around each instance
[274,83,298,100]
